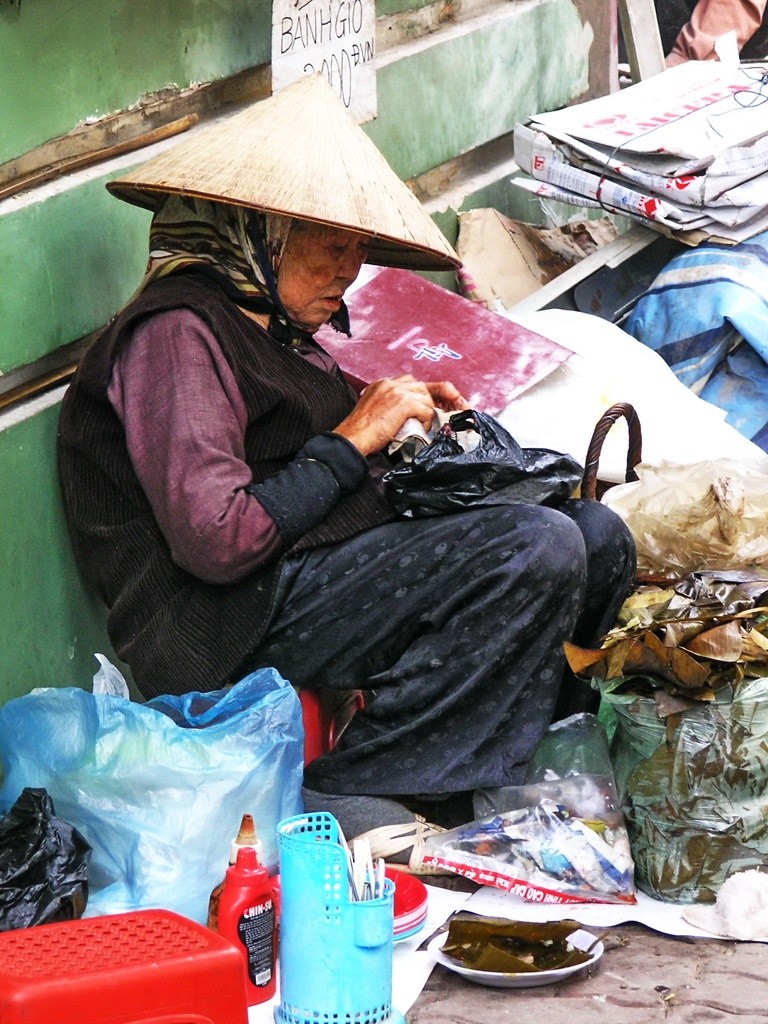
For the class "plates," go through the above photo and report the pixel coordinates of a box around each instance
[427,928,604,988]
[270,863,428,945]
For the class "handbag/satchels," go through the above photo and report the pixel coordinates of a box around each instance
[384,407,581,519]
[0,667,305,927]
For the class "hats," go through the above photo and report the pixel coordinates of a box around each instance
[105,71,463,272]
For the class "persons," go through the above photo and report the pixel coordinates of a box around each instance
[61,71,636,873]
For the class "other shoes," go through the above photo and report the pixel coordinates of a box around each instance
[300,787,461,876]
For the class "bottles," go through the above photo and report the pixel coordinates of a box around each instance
[229,814,261,867]
[207,847,278,1007]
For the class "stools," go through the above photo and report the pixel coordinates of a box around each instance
[298,689,366,767]
[0,908,249,1024]
